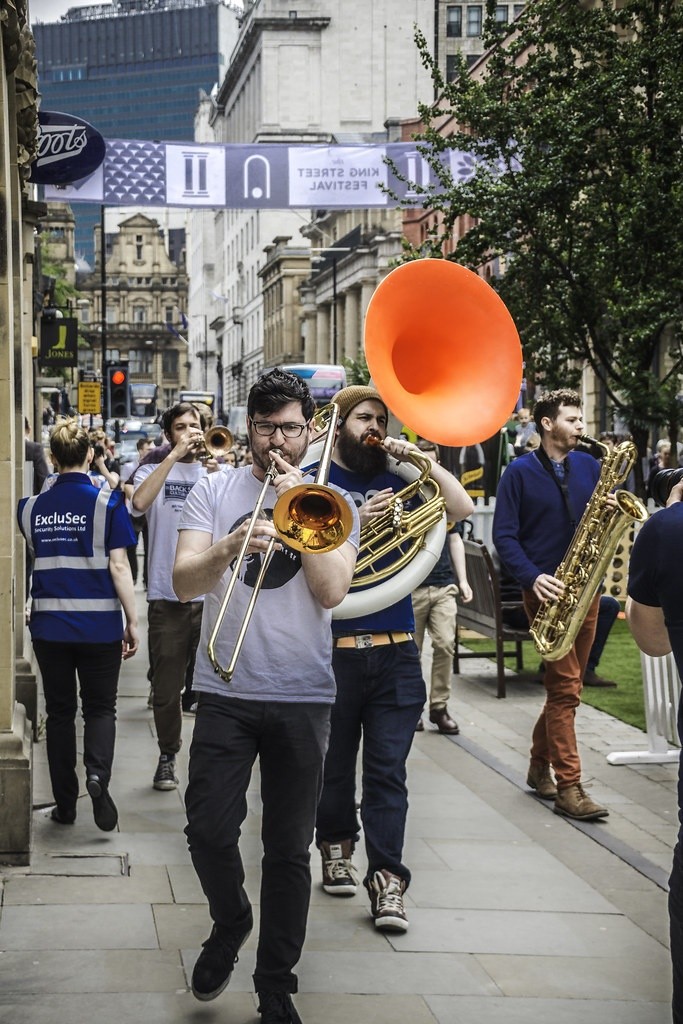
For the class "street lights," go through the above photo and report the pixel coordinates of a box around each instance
[192,313,207,392]
[309,256,337,364]
[147,340,158,383]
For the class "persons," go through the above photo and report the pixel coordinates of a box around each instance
[492,388,616,820]
[511,406,683,499]
[171,370,359,1024]
[17,385,475,831]
[625,475,683,1024]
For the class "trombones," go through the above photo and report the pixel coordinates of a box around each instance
[207,403,354,684]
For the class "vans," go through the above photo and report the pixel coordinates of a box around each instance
[228,406,247,435]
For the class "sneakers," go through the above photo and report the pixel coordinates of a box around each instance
[256,992,303,1024]
[321,840,359,895]
[191,921,252,1002]
[526,762,557,798]
[553,782,609,820]
[153,753,179,790]
[365,870,409,930]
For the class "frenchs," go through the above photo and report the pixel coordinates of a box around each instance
[295,257,524,620]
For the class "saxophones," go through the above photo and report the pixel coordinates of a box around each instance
[529,434,651,661]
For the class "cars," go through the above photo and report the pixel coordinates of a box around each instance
[106,419,164,464]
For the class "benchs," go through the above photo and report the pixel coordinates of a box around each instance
[450,539,534,700]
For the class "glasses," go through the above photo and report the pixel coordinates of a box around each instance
[249,417,312,438]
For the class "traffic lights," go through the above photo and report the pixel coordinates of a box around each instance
[107,366,132,419]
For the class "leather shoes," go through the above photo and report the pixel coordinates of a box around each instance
[415,718,425,730]
[429,705,459,732]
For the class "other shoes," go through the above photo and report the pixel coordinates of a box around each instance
[583,669,617,687]
[51,806,74,824]
[86,774,118,832]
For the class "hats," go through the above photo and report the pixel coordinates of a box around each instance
[330,385,386,422]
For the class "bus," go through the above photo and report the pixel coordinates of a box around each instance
[128,383,160,424]
[263,364,346,409]
[173,392,217,420]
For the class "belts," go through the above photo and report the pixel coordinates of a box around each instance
[337,631,414,649]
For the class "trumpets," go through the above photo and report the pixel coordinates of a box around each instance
[196,426,233,457]
